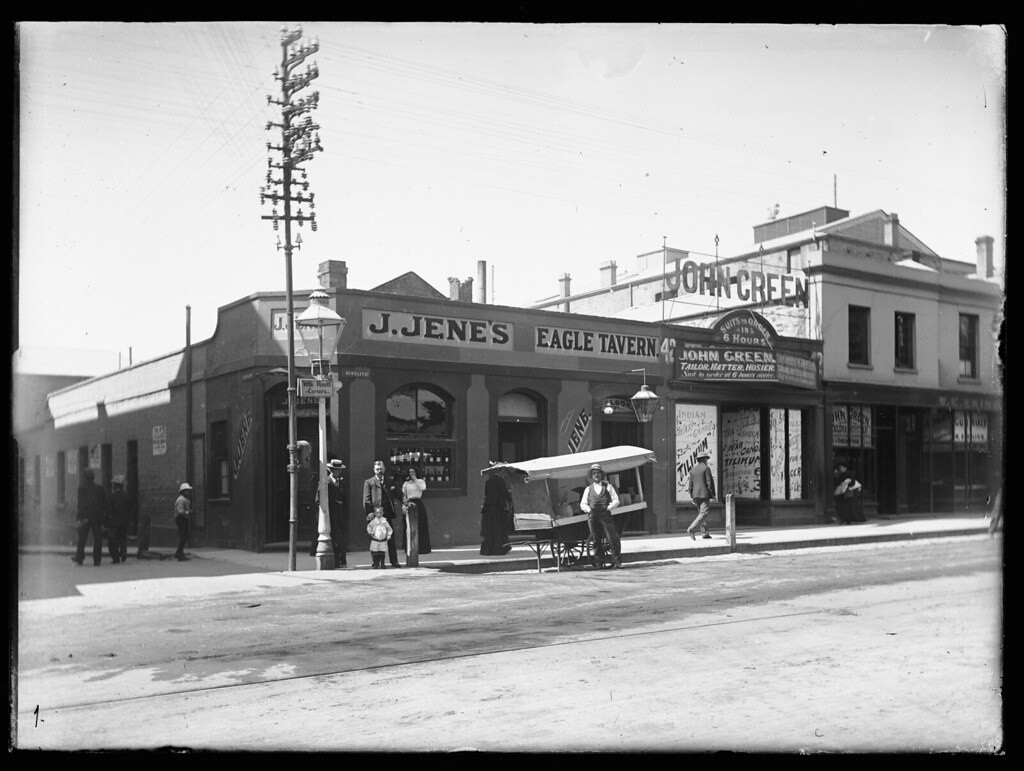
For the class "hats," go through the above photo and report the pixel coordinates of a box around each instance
[179,483,194,491]
[694,452,711,459]
[327,459,347,469]
[588,464,605,479]
[111,475,125,483]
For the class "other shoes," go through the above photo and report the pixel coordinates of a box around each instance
[393,563,401,568]
[613,561,624,569]
[703,534,712,539]
[687,530,696,541]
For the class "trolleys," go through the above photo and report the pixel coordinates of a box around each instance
[479,445,656,573]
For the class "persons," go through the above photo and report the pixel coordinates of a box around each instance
[480,462,514,556]
[580,464,627,569]
[103,475,130,564]
[401,466,431,554]
[686,451,716,541]
[367,505,393,569]
[988,484,1003,538]
[362,461,417,568]
[315,459,347,569]
[833,463,855,526]
[71,468,107,567]
[175,483,194,561]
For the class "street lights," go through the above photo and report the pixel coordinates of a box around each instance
[294,286,348,572]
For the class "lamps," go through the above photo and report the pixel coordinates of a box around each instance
[625,368,660,424]
